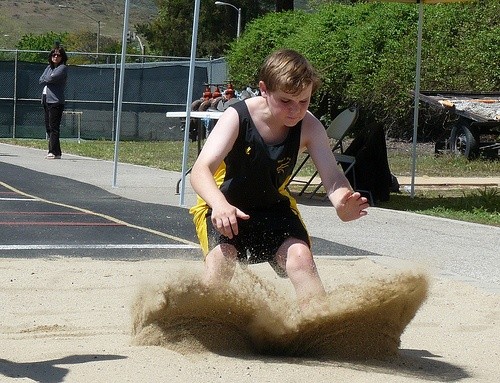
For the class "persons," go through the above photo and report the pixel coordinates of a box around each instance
[40,46,67,159]
[190,47,369,314]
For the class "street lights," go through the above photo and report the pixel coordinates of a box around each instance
[58,4,101,63]
[214,1,241,44]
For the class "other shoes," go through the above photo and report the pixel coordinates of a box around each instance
[44,153,62,159]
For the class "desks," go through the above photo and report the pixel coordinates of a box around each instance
[166,112,224,195]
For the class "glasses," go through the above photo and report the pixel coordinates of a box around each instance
[52,53,61,58]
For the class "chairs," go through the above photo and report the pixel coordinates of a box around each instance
[285,106,359,201]
